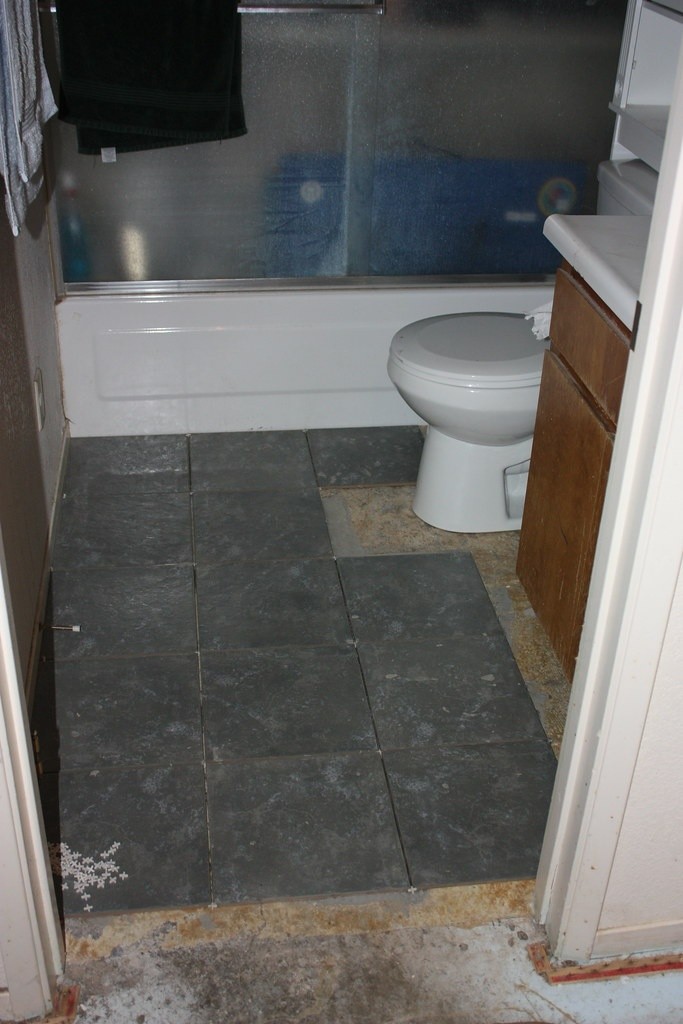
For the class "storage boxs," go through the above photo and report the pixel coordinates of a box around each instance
[609,0,683,173]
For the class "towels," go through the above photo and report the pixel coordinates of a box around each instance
[54,0,249,156]
[0,0,59,237]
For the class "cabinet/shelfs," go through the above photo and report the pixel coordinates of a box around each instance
[516,259,635,682]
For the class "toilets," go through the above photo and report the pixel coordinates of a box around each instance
[386,309,552,535]
